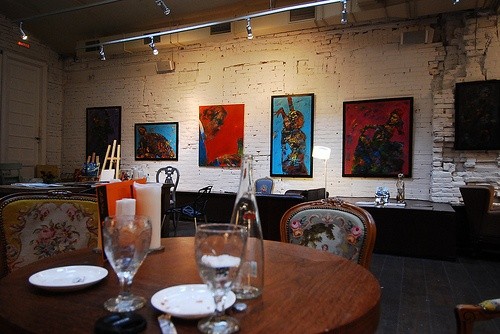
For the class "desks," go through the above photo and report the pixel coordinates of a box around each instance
[0,182,108,194]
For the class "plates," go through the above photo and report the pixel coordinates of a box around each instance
[150,283,237,320]
[28,265,108,291]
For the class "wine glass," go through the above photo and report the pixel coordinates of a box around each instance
[195,222,249,333]
[102,212,153,311]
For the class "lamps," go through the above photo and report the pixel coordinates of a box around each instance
[341,0,348,24]
[98,45,106,61]
[18,21,29,41]
[155,0,170,16]
[149,37,159,56]
[246,18,253,39]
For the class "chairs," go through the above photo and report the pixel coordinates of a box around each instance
[279,199,377,270]
[155,165,212,236]
[0,191,99,280]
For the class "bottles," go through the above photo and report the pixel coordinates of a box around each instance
[224,154,265,300]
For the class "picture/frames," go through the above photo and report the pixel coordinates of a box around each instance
[86,105,121,170]
[270,94,315,179]
[199,103,245,167]
[342,95,414,178]
[134,121,179,161]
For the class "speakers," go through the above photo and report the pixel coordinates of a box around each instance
[401,30,428,44]
[155,60,173,72]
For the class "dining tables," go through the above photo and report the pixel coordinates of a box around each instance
[0,237,380,334]
